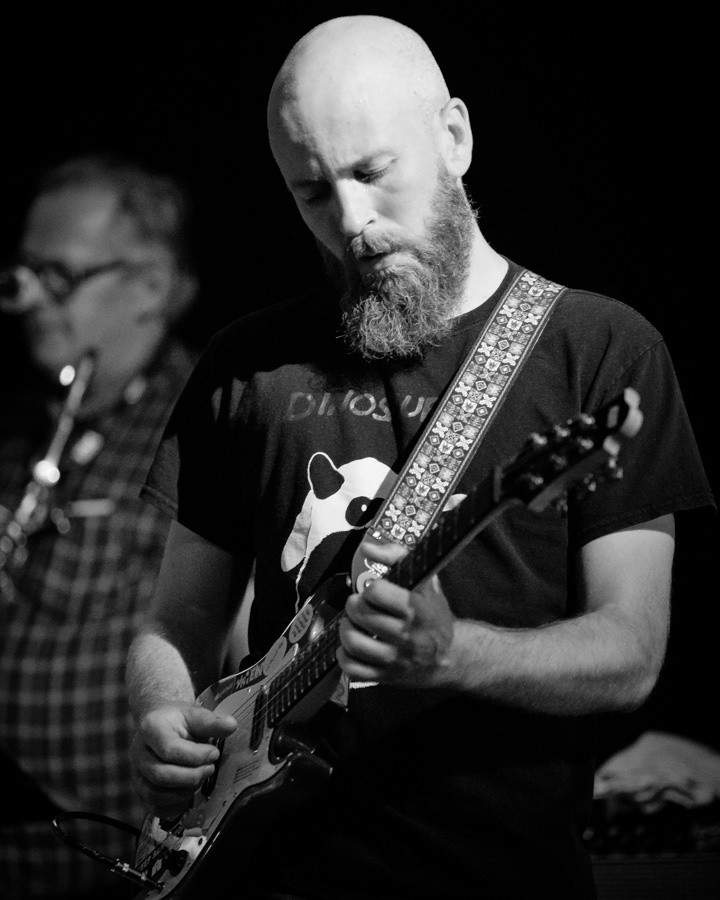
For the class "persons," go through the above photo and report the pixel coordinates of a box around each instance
[125,16,707,900]
[0,156,231,900]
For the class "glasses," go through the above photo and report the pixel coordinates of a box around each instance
[0,258,127,311]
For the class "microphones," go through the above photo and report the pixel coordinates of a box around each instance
[0,264,42,312]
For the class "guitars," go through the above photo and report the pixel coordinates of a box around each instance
[131,387,645,900]
